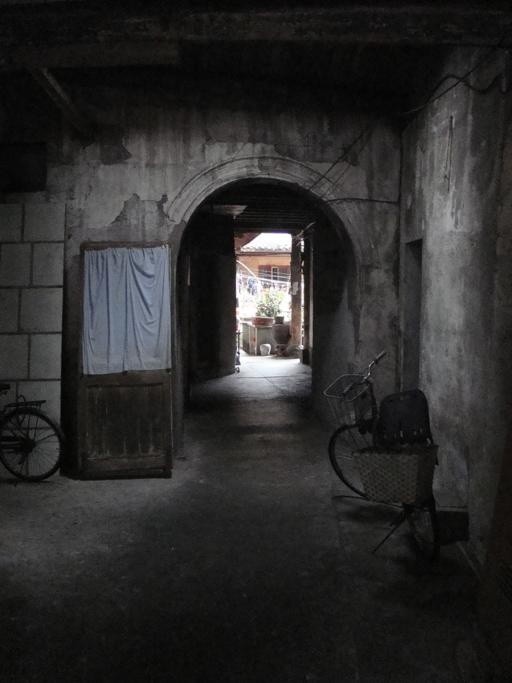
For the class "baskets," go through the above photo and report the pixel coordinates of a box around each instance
[322,373,372,427]
[351,445,438,506]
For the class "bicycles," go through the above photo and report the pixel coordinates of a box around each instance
[0,383,66,488]
[324,349,443,557]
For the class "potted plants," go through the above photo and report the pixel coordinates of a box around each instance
[251,291,284,326]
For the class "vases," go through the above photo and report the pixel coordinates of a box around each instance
[275,316,284,324]
